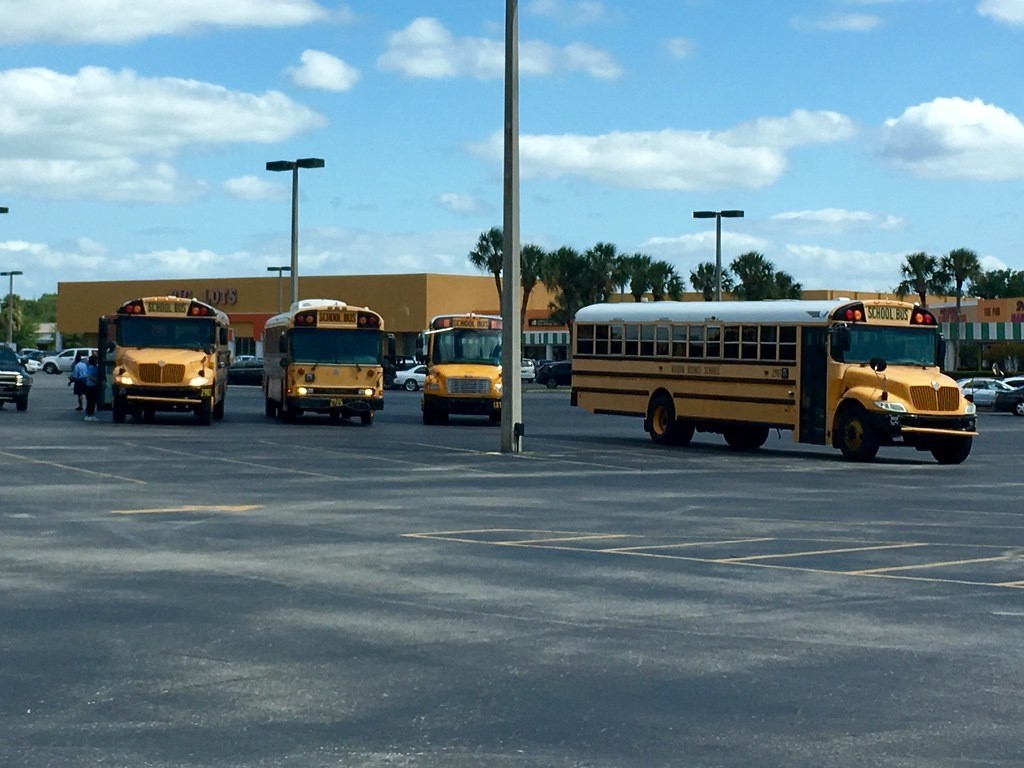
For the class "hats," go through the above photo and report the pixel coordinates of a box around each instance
[75,355,82,360]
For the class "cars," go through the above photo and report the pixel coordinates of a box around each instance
[521,358,536,383]
[383,359,427,392]
[15,348,46,374]
[535,361,571,390]
[991,386,1024,417]
[956,377,1024,407]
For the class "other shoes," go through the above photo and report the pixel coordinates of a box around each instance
[84,416,100,421]
[75,406,83,410]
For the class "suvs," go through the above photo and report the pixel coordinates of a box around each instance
[41,348,99,375]
[0,345,33,411]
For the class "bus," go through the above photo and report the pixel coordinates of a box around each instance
[97,295,231,427]
[416,313,503,426]
[263,298,396,426]
[570,299,979,465]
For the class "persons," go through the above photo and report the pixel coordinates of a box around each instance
[84,355,100,421]
[990,363,1004,377]
[68,356,87,411]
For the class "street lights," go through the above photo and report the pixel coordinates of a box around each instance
[1,270,23,344]
[266,158,325,304]
[693,210,744,301]
[267,267,293,314]
